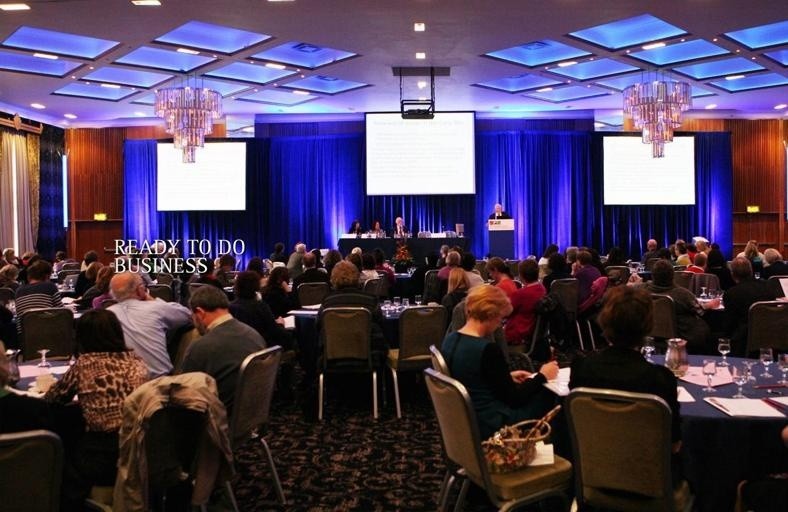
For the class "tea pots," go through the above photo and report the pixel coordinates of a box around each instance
[662,337,689,378]
[4,348,22,385]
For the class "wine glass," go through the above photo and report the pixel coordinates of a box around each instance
[709,289,715,299]
[62,278,74,291]
[775,354,788,385]
[699,286,708,300]
[402,298,409,311]
[701,358,717,393]
[759,347,774,378]
[716,338,731,366]
[35,348,52,377]
[383,300,392,318]
[729,362,749,398]
[407,266,416,278]
[414,295,422,307]
[639,335,656,364]
[393,296,401,313]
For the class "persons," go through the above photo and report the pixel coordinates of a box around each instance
[443,283,567,449]
[566,282,683,490]
[1,216,787,430]
[731,425,786,508]
[41,307,151,512]
[148,283,269,510]
[487,198,512,220]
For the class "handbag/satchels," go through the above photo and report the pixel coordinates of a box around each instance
[480,405,561,474]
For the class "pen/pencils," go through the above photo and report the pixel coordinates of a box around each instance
[709,398,729,412]
[753,384,786,389]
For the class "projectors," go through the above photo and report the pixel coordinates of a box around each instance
[400,99,436,120]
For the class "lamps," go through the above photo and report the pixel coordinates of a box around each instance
[624,67,693,160]
[155,69,221,164]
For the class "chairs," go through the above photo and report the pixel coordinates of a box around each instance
[444,231,456,237]
[418,230,431,238]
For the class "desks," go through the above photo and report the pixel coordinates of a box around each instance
[339,237,474,268]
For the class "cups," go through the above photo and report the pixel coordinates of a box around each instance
[637,267,644,273]
